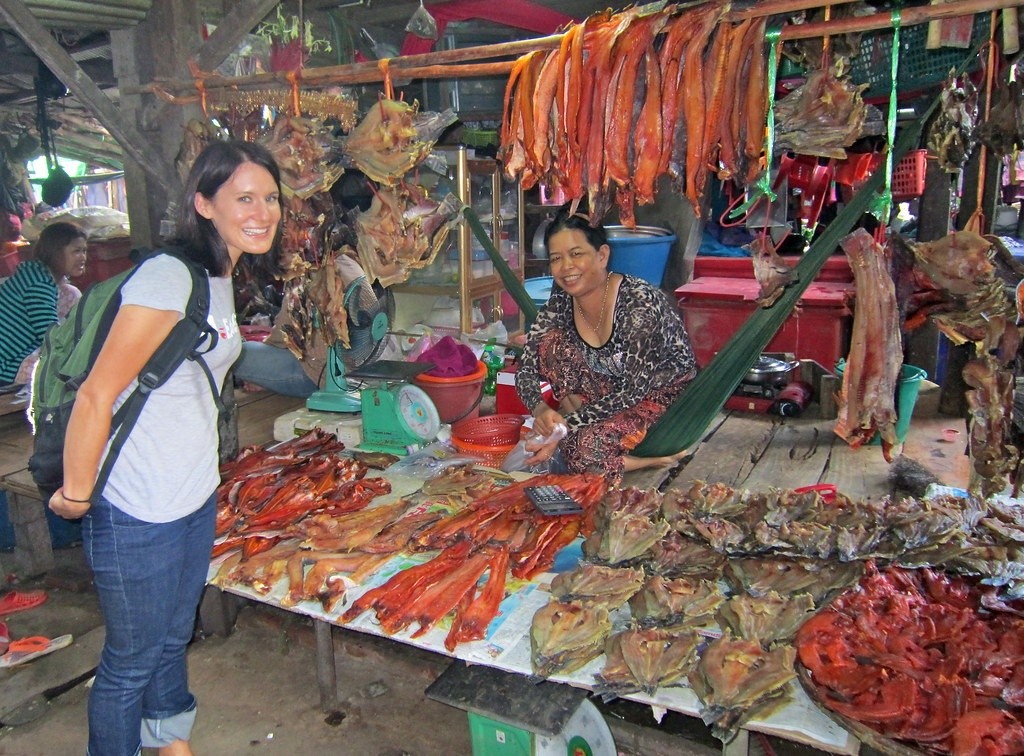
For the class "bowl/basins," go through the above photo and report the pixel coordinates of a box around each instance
[942,428,960,442]
[603,226,670,236]
[607,235,677,289]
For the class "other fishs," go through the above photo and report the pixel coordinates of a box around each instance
[184,97,459,360]
[210,426,1024,756]
[833,227,904,465]
[773,56,869,160]
[496,3,770,232]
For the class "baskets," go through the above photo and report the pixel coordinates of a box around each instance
[853,149,927,199]
[451,412,525,469]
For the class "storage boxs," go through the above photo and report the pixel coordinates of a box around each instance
[845,10,1000,99]
[688,253,856,284]
[673,276,857,374]
[839,148,928,203]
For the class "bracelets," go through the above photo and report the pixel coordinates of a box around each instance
[62,492,89,503]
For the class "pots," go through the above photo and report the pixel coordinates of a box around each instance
[744,356,800,383]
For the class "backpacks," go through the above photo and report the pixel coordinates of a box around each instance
[27,245,228,491]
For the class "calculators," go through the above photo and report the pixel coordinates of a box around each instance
[523,484,584,517]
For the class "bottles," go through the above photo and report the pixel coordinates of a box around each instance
[477,337,505,396]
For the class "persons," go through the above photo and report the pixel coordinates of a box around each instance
[48,138,285,756]
[231,254,379,399]
[514,209,697,479]
[811,182,877,250]
[0,222,88,387]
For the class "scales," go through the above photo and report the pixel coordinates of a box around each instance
[342,360,441,455]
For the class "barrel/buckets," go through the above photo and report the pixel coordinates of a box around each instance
[834,358,928,445]
[412,360,485,424]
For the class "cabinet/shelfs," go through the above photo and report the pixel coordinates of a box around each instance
[392,143,525,340]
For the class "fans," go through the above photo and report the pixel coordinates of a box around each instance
[306,273,397,415]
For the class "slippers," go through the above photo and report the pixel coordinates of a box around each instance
[0,589,48,616]
[0,623,11,655]
[0,634,73,669]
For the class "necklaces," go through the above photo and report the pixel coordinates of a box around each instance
[578,271,613,331]
